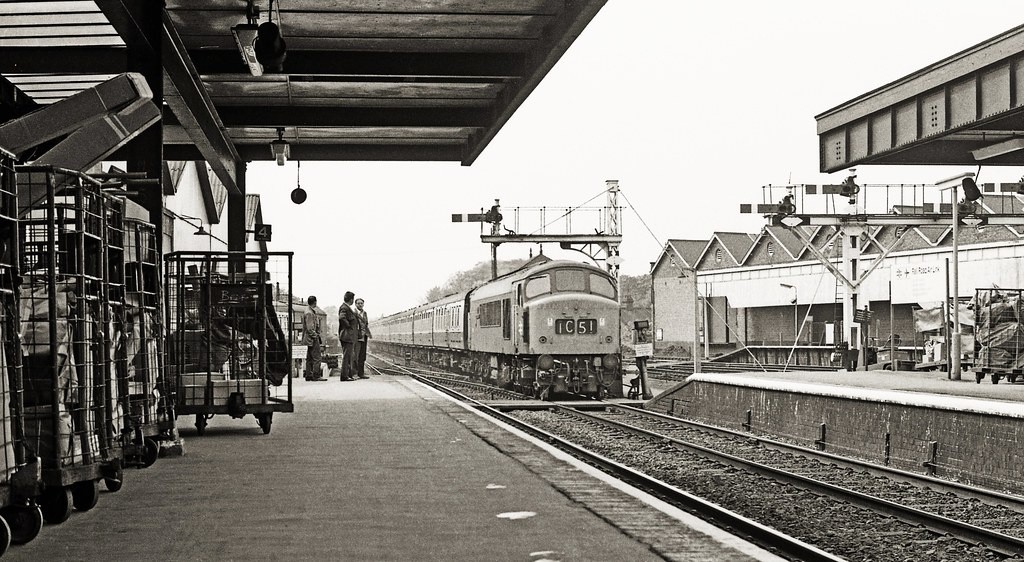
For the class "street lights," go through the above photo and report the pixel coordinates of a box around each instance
[779,283,798,340]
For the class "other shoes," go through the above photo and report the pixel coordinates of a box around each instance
[357,374,369,379]
[315,377,327,381]
[306,378,315,381]
[342,377,356,381]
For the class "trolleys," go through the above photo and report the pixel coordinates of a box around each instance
[0,144,295,557]
[973,286,1024,385]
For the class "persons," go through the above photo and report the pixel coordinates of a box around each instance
[302,296,327,381]
[352,299,372,379]
[339,292,362,381]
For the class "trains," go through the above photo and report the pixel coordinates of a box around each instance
[367,259,621,402]
